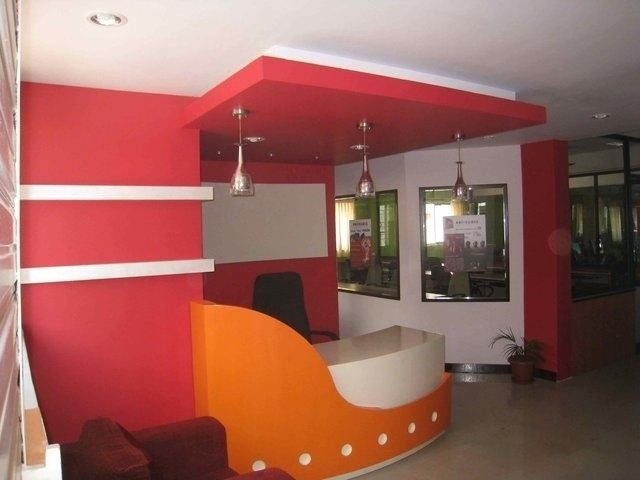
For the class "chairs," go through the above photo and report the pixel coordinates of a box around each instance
[56,412,297,480]
[338,254,396,286]
[252,271,341,346]
[572,233,640,293]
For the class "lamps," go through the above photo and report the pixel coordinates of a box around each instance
[452,133,471,202]
[354,120,378,201]
[229,108,258,199]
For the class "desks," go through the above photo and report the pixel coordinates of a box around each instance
[423,265,505,299]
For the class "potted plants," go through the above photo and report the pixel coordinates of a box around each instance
[487,325,544,384]
